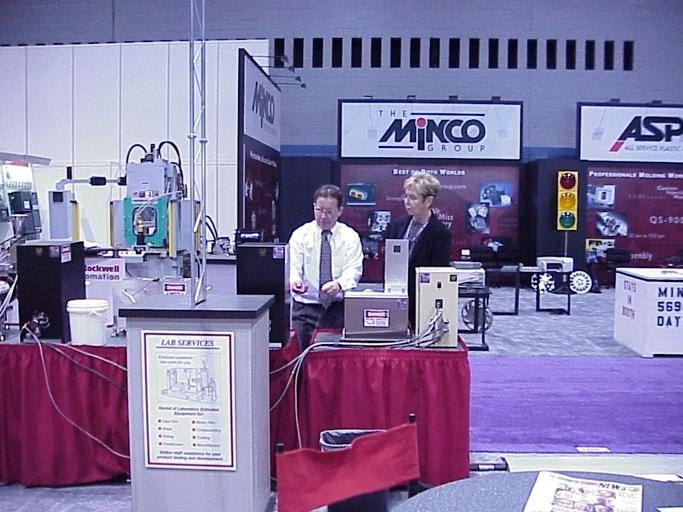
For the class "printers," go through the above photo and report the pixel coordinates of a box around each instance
[536,257,574,273]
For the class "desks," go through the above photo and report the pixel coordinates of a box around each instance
[1,330,284,488]
[306,331,469,487]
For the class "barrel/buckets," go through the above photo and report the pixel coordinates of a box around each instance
[66,299,109,345]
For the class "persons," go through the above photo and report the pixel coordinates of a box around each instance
[288,184,363,359]
[381,171,452,333]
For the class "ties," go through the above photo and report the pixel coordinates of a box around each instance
[319,229,335,309]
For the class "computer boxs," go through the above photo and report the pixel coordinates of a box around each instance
[416,266,459,347]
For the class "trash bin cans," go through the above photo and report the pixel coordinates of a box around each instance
[320,427,389,512]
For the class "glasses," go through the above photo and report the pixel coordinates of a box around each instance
[313,206,339,214]
[402,194,426,202]
